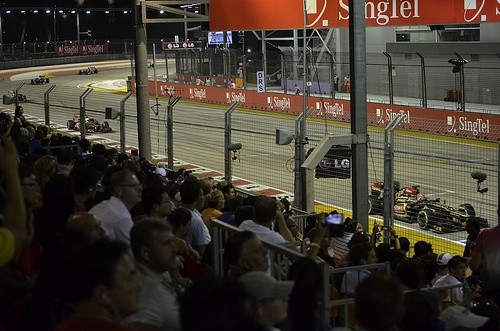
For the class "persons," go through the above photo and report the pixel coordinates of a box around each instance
[343,74,350,93]
[0,105,500,331]
[239,63,243,70]
[267,73,272,86]
[277,71,281,86]
[333,74,339,91]
[444,89,456,102]
[294,84,300,95]
[204,77,210,83]
[195,76,203,85]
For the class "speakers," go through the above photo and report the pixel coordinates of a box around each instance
[3,95,13,105]
[275,128,294,145]
[104,107,119,120]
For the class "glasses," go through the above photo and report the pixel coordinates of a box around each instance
[157,197,172,205]
[20,182,40,191]
[120,180,143,189]
[454,265,467,270]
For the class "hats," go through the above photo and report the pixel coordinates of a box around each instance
[436,252,452,266]
[228,271,294,299]
[438,300,489,331]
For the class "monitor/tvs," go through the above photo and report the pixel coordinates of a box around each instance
[324,213,343,225]
[207,31,233,45]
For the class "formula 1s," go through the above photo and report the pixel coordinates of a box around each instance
[367,180,489,235]
[78,66,99,75]
[30,75,50,85]
[66,113,112,134]
[7,90,27,103]
